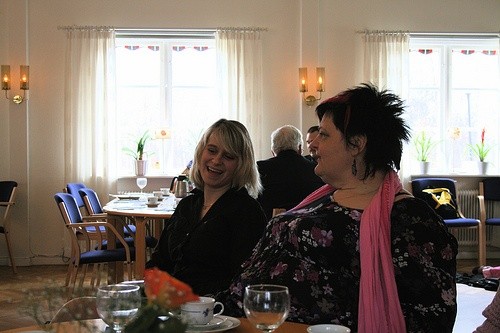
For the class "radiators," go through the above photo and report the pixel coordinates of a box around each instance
[447,189,500,249]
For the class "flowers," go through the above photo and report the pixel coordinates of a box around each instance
[471,128,491,162]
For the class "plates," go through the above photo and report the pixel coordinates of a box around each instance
[184,316,241,333]
[183,315,228,330]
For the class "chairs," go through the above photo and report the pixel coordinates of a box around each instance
[0,180,19,275]
[408,177,483,265]
[54,183,159,301]
[479,175,500,265]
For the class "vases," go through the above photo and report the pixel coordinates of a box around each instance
[477,161,488,175]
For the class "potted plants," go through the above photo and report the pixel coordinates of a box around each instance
[133,133,147,176]
[411,132,431,176]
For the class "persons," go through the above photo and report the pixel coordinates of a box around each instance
[305,126,319,161]
[204,84,457,333]
[256,126,323,220]
[49,118,266,324]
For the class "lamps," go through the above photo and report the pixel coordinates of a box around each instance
[0,64,30,105]
[298,67,324,106]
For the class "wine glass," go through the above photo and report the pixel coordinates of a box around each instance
[96,281,142,333]
[136,178,148,197]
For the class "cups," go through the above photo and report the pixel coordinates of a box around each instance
[306,324,351,333]
[242,284,291,333]
[148,197,158,205]
[178,296,224,325]
[160,188,170,195]
[154,192,163,199]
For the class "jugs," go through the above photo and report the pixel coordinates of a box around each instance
[169,174,189,198]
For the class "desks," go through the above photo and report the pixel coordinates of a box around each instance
[102,198,178,280]
[0,319,310,333]
